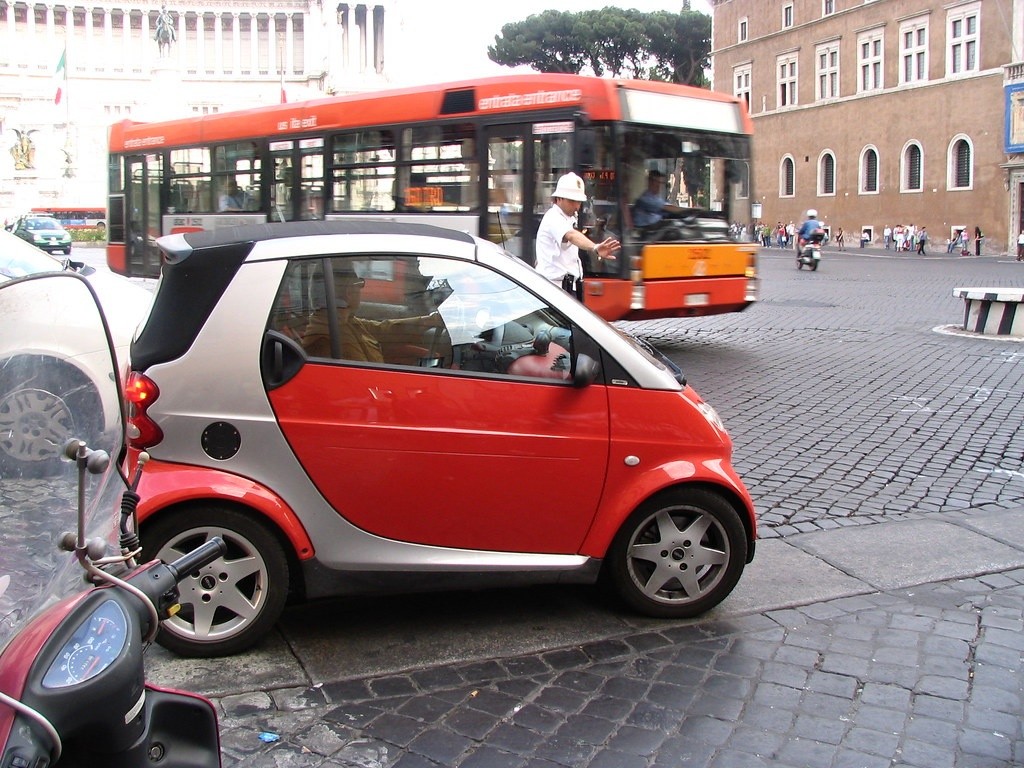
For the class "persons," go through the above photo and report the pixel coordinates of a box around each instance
[959,228,969,251]
[974,227,981,256]
[1016,230,1024,261]
[536,172,621,302]
[152,4,176,42]
[837,228,846,251]
[218,176,252,211]
[860,230,870,248]
[946,229,960,253]
[771,221,795,249]
[633,170,692,227]
[728,220,745,238]
[301,260,444,364]
[883,224,930,255]
[751,220,772,248]
[795,210,820,258]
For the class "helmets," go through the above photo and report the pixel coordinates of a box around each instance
[550,171,587,202]
[807,209,817,217]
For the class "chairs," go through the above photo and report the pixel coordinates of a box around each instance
[276,286,308,347]
[130,180,325,222]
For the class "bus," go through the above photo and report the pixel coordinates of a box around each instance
[29,206,106,240]
[106,73,761,325]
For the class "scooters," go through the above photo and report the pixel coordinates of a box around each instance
[793,226,826,272]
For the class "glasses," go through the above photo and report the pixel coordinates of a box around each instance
[352,278,365,289]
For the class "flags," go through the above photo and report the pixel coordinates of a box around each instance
[55,53,66,105]
[282,89,286,103]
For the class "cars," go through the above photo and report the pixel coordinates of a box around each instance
[95,220,760,659]
[1,228,158,478]
[11,216,73,255]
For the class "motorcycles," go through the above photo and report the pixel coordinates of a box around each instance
[0,273,224,768]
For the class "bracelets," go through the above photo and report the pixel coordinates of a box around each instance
[594,245,599,257]
